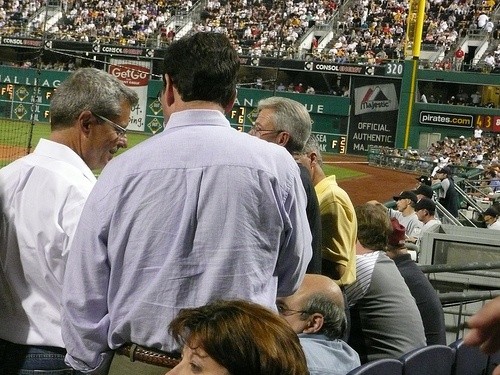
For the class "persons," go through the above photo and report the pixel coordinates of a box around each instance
[386,218,446,347]
[458,295,500,374]
[167,299,308,375]
[343,204,426,366]
[63,31,312,375]
[292,132,358,344]
[275,273,360,375]
[0,0,499,253]
[0,66,140,375]
[247,97,324,273]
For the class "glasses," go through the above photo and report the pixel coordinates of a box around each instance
[75,110,127,138]
[252,122,284,134]
[277,304,313,315]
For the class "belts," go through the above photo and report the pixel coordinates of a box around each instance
[117,343,181,368]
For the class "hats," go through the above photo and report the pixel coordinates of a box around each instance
[416,175,432,186]
[483,208,497,217]
[393,191,418,204]
[387,217,406,246]
[412,186,434,199]
[411,198,436,212]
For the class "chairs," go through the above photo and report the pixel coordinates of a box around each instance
[346,339,500,375]
